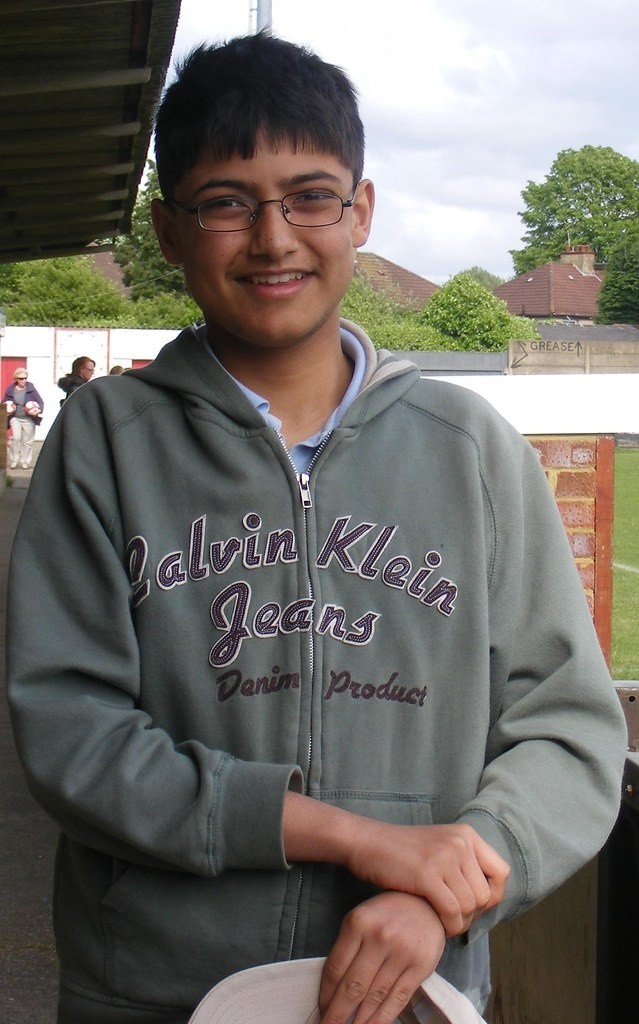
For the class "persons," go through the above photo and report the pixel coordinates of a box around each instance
[4,24,628,1024]
[0,368,43,469]
[59,356,95,408]
[108,365,124,374]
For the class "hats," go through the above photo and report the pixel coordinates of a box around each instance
[188,955,486,1023]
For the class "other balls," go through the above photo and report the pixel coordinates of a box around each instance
[3,400,17,415]
[24,400,40,416]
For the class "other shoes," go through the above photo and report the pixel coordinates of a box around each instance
[11,463,17,469]
[22,463,28,470]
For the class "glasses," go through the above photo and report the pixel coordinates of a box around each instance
[171,183,361,233]
[84,367,94,373]
[16,378,28,381]
